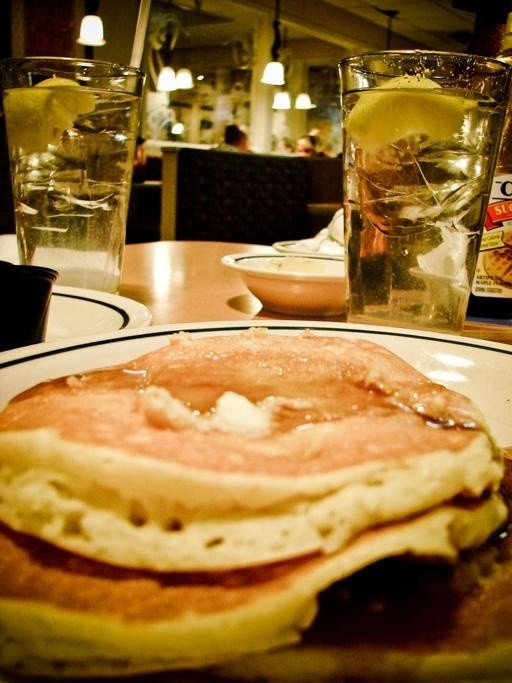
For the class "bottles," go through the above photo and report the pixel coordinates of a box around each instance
[465,10,511,325]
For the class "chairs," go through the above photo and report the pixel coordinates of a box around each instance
[159,143,312,242]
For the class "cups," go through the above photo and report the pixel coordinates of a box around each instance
[339,51,510,337]
[0,57,147,296]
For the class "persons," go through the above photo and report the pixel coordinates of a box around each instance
[209,125,254,154]
[272,135,331,159]
[130,136,148,183]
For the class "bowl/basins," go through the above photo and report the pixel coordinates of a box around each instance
[221,253,346,315]
[0,261,58,352]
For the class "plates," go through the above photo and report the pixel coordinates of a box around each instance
[46,285,154,342]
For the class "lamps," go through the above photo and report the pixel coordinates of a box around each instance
[74,0,108,48]
[258,0,318,111]
[155,23,196,93]
